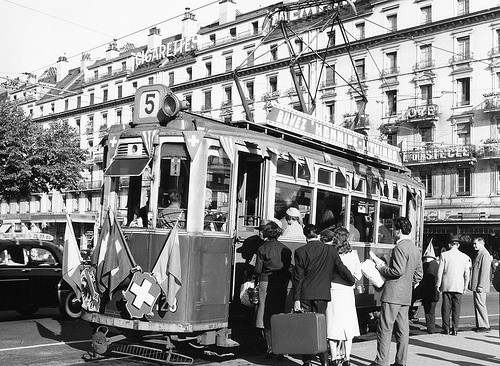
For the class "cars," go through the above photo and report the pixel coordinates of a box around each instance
[0,239,85,320]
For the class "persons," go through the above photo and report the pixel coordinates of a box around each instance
[409,260,441,334]
[325,227,363,366]
[135,189,395,244]
[369,216,424,366]
[294,224,357,366]
[469,237,493,332]
[251,221,292,361]
[435,235,469,336]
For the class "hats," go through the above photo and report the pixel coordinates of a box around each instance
[423,239,436,260]
[447,235,461,243]
[285,206,301,217]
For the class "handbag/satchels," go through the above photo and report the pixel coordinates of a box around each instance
[248,286,261,304]
[240,280,260,309]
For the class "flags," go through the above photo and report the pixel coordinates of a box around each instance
[90,210,111,294]
[62,220,84,299]
[151,226,181,307]
[101,216,134,300]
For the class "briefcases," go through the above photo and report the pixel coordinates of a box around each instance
[271,307,327,355]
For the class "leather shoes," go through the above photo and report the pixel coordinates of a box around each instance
[342,360,350,366]
[369,362,380,366]
[302,362,312,366]
[329,361,337,366]
[441,330,449,334]
[452,330,457,335]
[390,362,403,366]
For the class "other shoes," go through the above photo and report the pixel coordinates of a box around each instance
[476,328,491,332]
[471,327,482,330]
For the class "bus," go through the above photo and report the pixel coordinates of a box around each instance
[79,94,425,366]
